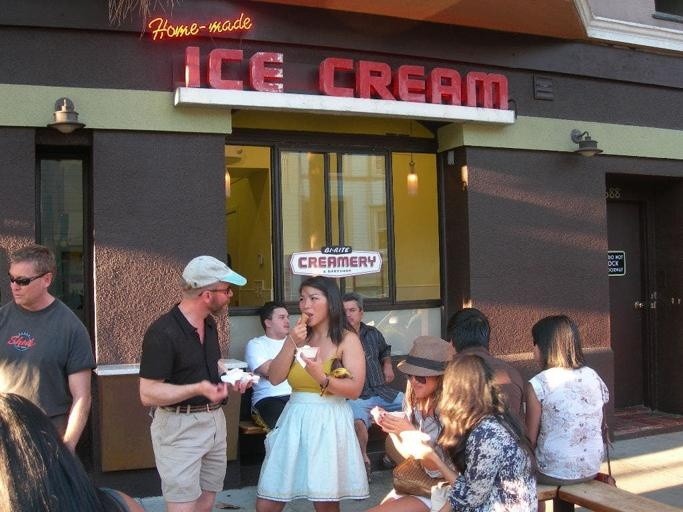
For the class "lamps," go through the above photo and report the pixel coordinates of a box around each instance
[47,97,86,133]
[572,128,603,158]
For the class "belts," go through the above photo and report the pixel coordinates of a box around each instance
[159,402,226,415]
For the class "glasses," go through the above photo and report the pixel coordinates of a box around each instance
[7,271,50,286]
[404,374,427,384]
[199,286,234,298]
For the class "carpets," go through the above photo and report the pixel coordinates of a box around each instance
[612,406,683,438]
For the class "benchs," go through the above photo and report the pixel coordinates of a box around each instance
[239,420,385,460]
[559,480,683,512]
[535,484,558,512]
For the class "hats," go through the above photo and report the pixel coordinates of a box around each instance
[397,337,458,378]
[182,255,249,289]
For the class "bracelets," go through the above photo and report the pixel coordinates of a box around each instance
[320,372,330,398]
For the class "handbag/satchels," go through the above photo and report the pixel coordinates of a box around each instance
[332,359,345,378]
[594,375,618,488]
[391,456,454,501]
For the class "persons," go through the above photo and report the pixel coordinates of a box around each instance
[139,255,247,512]
[528,315,610,485]
[436,357,541,512]
[0,245,97,453]
[255,277,371,511]
[0,392,150,512]
[245,304,315,433]
[324,291,419,483]
